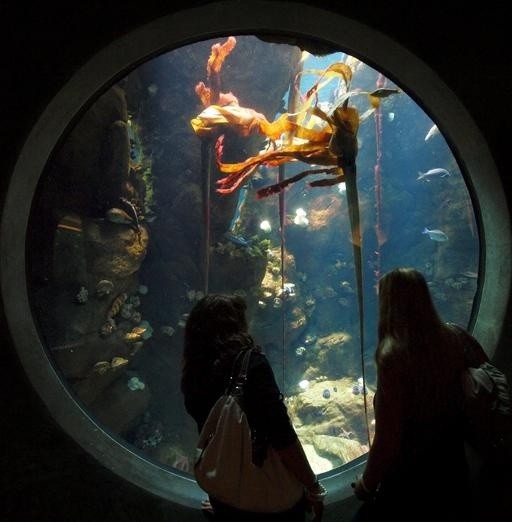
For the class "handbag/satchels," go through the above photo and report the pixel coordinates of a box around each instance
[360,474,373,495]
[304,482,327,502]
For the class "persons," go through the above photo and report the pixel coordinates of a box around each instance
[350,269,511,521]
[179,291,327,521]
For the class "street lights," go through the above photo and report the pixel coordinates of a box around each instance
[191,397,308,515]
[459,361,512,457]
